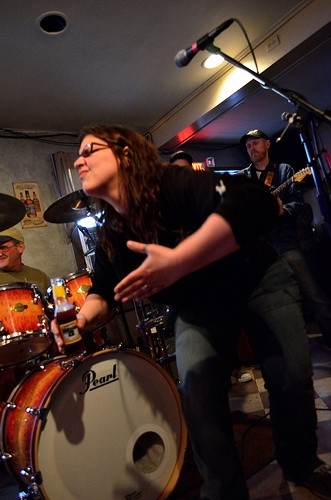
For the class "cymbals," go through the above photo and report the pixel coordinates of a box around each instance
[0,192,26,232]
[43,189,105,224]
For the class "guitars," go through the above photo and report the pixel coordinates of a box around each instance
[271,166,312,196]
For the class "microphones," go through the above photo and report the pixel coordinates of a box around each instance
[174,16,234,68]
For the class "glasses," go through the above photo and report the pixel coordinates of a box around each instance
[76,143,106,159]
[0,244,15,251]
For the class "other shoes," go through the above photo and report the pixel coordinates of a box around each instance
[302,463,331,499]
[235,371,252,383]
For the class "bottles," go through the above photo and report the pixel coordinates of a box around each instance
[50,277,86,357]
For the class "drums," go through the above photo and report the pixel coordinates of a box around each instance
[46,268,119,335]
[0,344,187,500]
[0,282,54,370]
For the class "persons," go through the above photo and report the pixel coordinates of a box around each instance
[72,123,330,500]
[0,224,55,340]
[168,150,194,169]
[238,127,328,342]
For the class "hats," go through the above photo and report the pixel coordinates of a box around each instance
[0,227,24,243]
[240,130,268,147]
